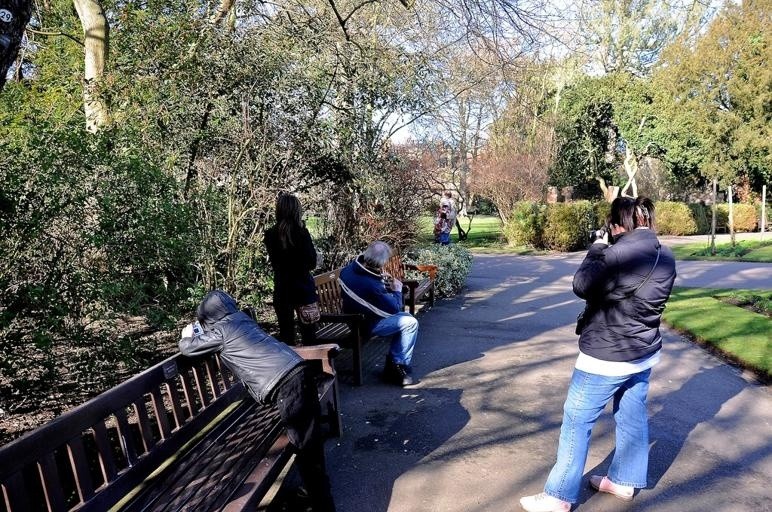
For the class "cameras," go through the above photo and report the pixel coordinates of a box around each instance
[192,321,204,337]
[589,226,616,245]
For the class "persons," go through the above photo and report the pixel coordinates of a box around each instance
[263,194,321,346]
[433,190,457,246]
[337,242,418,387]
[518,196,677,512]
[176,291,337,512]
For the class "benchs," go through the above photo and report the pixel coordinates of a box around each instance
[295,263,409,385]
[383,246,438,316]
[0,345,342,512]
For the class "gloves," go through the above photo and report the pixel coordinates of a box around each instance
[592,231,608,244]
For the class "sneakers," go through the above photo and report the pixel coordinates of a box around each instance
[590,474,634,502]
[520,493,571,512]
[392,365,412,384]
[386,356,396,371]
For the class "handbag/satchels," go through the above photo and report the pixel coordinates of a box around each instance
[575,246,662,334]
[298,277,320,324]
